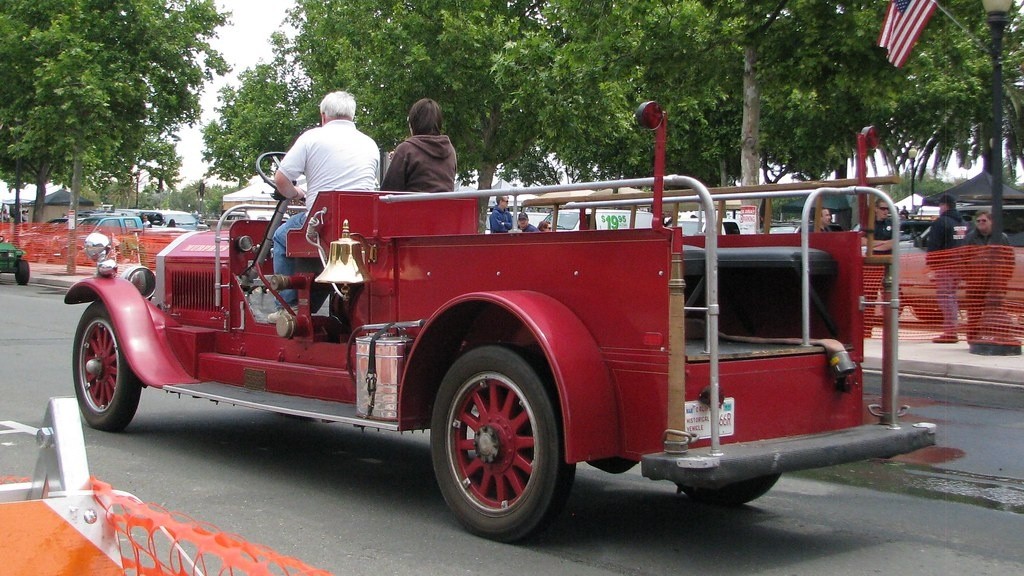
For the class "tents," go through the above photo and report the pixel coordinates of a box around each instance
[921,169,1024,215]
[780,194,852,214]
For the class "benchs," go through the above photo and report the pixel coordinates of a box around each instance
[683,244,840,337]
[282,192,480,259]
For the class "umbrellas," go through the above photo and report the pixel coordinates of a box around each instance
[529,188,646,211]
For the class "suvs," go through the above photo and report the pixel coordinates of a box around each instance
[664,218,744,234]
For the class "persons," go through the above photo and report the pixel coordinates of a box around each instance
[22,210,28,222]
[167,219,176,228]
[380,98,457,193]
[899,205,910,218]
[268,92,380,324]
[489,196,513,234]
[809,208,832,233]
[142,215,150,228]
[926,193,969,344]
[860,199,893,338]
[538,221,551,232]
[517,212,538,233]
[960,210,1015,345]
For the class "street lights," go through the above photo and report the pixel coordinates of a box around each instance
[968,1,1021,356]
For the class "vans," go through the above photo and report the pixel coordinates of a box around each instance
[539,208,656,230]
[485,208,549,234]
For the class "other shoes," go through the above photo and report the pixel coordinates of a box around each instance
[933,334,958,344]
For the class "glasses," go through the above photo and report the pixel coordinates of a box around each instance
[976,219,988,223]
[549,227,551,230]
[878,207,888,211]
[504,200,508,203]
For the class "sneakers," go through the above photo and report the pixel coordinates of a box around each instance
[268,306,298,323]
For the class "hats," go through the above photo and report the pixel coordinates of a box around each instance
[518,212,528,220]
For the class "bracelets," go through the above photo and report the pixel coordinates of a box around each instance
[292,191,298,198]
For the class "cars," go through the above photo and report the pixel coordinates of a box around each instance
[763,203,1024,316]
[117,208,199,230]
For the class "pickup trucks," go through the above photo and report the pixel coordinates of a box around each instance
[21,209,185,259]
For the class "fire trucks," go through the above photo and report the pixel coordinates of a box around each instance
[62,99,938,542]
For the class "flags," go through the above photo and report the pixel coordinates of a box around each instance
[876,0,937,69]
